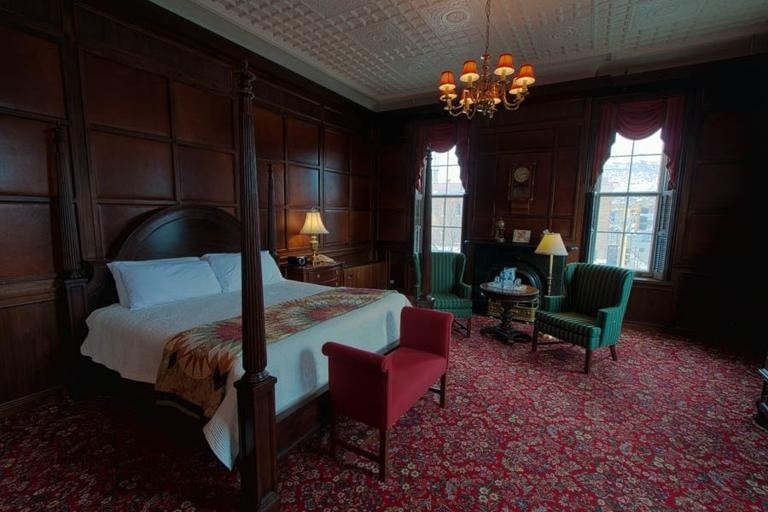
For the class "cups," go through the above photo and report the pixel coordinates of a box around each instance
[492,271,522,289]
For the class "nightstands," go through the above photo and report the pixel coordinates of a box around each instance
[282,257,344,287]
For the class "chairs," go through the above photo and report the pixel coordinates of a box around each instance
[532,263,634,373]
[419,253,472,337]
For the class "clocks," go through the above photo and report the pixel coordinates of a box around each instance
[510,166,532,199]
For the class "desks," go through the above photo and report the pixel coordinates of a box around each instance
[465,239,567,318]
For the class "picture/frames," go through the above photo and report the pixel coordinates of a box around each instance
[512,229,531,243]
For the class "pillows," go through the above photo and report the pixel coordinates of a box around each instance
[106,251,284,310]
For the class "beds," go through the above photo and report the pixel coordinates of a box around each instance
[88,206,404,473]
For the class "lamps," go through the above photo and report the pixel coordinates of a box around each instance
[299,208,336,267]
[534,233,569,295]
[438,0,538,120]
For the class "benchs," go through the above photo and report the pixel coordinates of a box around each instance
[322,307,455,482]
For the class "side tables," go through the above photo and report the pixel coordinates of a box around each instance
[479,282,540,344]
